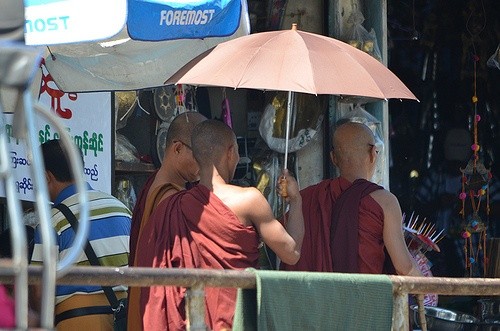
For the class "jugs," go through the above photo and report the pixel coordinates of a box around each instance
[477,298,500,331]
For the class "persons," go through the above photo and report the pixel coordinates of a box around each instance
[275,122,426,277]
[25,139,133,331]
[124,111,304,331]
[0,281,46,331]
[124,112,210,331]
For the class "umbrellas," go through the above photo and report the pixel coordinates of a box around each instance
[165,23,420,198]
[0,0,249,138]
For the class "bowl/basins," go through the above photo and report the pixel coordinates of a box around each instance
[410,305,485,331]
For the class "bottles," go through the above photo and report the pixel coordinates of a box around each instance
[113,178,133,212]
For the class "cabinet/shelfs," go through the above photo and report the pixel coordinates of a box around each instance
[230,135,272,188]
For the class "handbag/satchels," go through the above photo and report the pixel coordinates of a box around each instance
[113,298,128,331]
